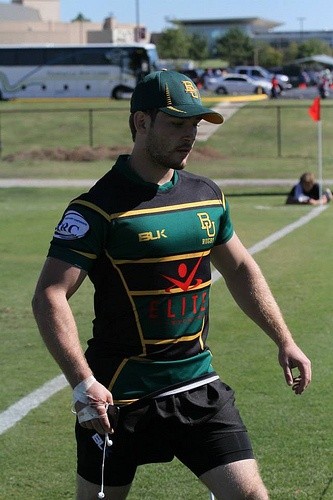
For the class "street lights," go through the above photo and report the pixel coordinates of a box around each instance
[72,12,92,41]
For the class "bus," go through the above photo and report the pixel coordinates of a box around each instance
[0,42,160,101]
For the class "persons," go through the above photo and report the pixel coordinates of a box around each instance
[195,65,333,99]
[284,173,333,205]
[32,69,312,500]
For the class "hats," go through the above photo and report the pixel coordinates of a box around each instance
[130,68,224,125]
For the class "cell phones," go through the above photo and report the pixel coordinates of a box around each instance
[106,404,119,428]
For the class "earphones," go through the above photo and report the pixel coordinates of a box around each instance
[97,492,105,498]
[105,434,112,446]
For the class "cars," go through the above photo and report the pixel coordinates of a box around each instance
[158,60,195,71]
[293,68,330,86]
[178,70,204,89]
[319,75,333,97]
[208,76,282,96]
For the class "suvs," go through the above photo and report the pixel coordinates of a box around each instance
[235,66,292,90]
